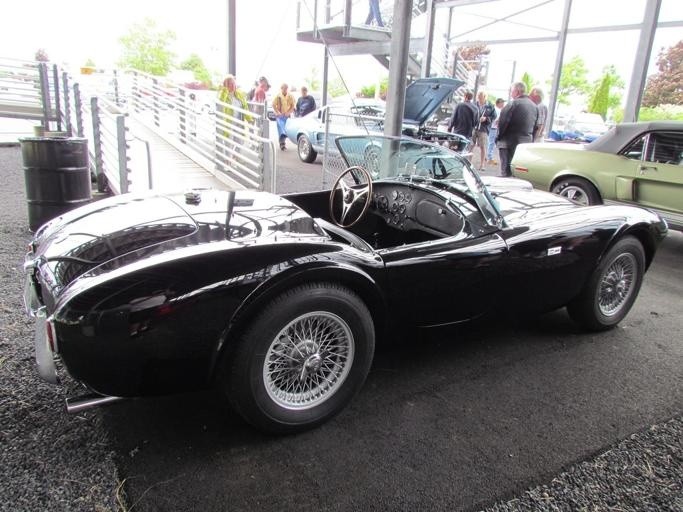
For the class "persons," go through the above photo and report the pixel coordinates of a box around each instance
[253,75,267,151]
[465,91,496,171]
[528,88,547,143]
[494,81,539,177]
[247,82,270,149]
[447,93,480,155]
[295,86,316,118]
[484,98,508,165]
[364,0,384,27]
[215,75,255,174]
[271,83,295,151]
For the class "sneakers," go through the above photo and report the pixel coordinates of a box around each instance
[479,159,498,170]
[280,143,286,150]
[225,162,238,171]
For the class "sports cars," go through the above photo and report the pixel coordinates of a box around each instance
[21,130,673,440]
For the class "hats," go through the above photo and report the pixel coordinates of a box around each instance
[496,98,507,104]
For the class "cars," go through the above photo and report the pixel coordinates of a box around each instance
[509,119,683,233]
[285,77,470,178]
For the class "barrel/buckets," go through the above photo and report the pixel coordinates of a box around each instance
[19,137,91,230]
